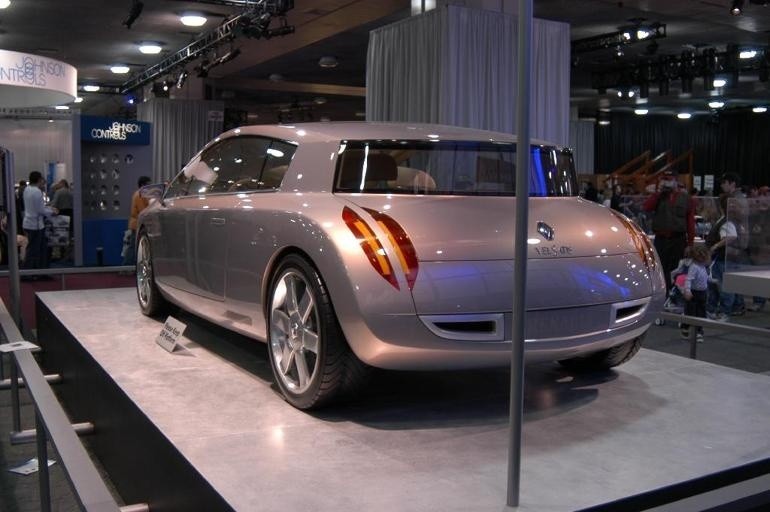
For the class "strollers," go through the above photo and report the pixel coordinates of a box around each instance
[653,247,730,328]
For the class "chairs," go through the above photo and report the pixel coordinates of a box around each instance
[231,153,438,194]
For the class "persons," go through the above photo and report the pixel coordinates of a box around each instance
[1,169,73,283]
[579,169,770,323]
[678,244,720,343]
[117,175,154,276]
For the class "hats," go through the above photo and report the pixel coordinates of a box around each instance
[661,168,679,176]
[715,171,739,181]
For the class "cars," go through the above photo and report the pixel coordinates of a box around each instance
[133,120,668,409]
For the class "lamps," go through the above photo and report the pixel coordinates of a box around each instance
[571,16,770,98]
[118,0,296,105]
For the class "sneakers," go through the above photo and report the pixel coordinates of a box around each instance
[678,304,762,343]
